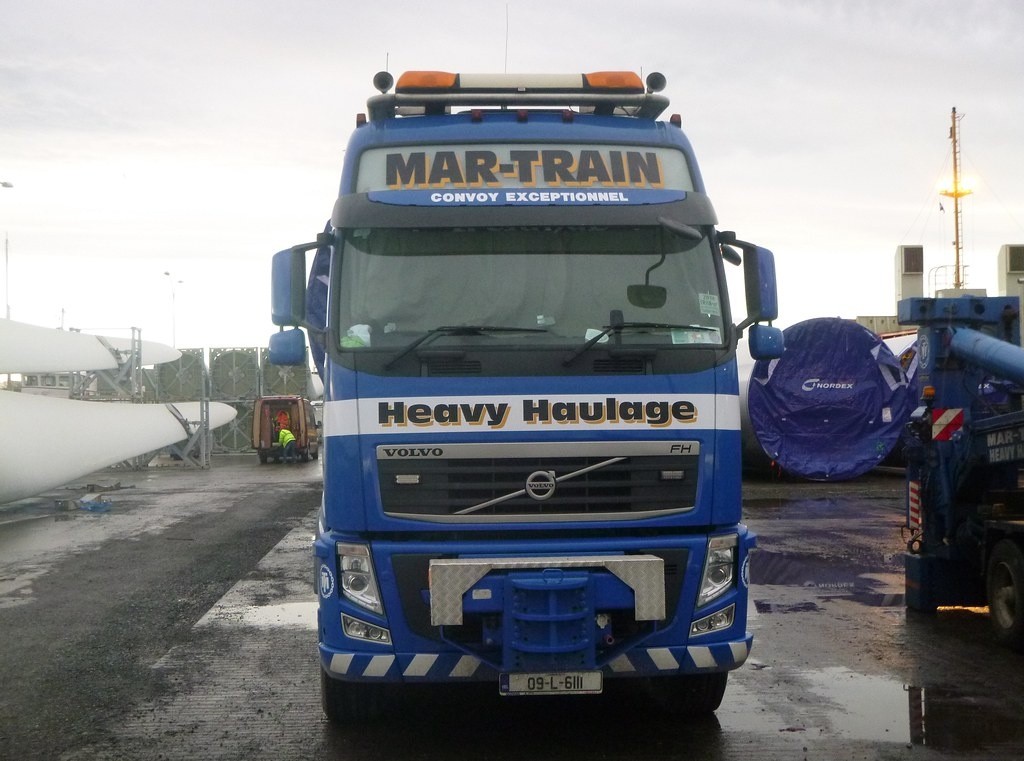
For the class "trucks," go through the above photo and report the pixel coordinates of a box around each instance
[270,67,784,723]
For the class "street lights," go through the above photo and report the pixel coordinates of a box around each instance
[163,270,186,353]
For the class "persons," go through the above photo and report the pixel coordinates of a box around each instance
[272,423,298,466]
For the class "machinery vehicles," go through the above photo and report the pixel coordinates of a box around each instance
[890,294,1024,648]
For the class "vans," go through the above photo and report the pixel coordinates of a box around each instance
[253,395,322,463]
